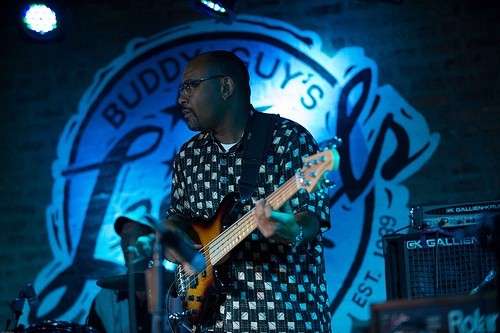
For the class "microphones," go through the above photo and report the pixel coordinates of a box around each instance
[25,282,39,309]
[193,0,236,26]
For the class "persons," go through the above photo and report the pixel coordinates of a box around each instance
[85,209,167,333]
[162,51,330,333]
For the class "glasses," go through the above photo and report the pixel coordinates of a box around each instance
[178,75,226,96]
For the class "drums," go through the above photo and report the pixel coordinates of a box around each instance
[22,319,93,333]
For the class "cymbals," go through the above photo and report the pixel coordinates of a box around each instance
[95,268,175,296]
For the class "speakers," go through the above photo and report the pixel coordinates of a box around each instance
[397,228,500,301]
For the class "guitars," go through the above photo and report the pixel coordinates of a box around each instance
[173,134,344,325]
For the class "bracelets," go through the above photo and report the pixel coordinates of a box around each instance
[291,223,304,248]
